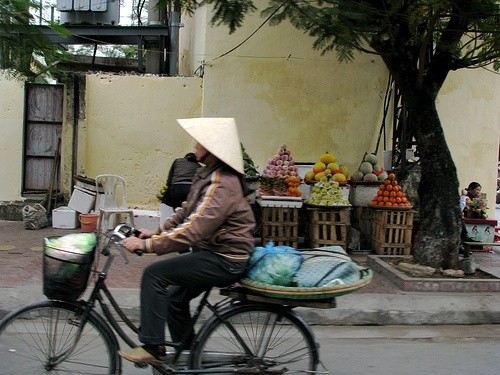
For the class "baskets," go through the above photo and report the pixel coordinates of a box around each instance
[43,235,96,302]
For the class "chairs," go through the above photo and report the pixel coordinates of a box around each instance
[95,174,134,236]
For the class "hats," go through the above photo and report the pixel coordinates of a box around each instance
[176,117,244,175]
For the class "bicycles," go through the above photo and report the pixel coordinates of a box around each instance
[0,224,330,375]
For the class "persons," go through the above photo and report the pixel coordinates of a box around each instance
[161,152,202,210]
[116,118,255,364]
[459,182,482,218]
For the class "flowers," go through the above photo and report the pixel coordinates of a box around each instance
[464,193,489,218]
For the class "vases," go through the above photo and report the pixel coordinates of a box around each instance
[463,219,497,251]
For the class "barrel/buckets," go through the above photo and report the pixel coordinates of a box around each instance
[78,214,99,232]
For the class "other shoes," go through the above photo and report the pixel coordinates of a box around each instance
[117,346,165,367]
[158,347,168,360]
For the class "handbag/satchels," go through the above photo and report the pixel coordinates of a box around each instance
[248,241,303,286]
[43,232,96,276]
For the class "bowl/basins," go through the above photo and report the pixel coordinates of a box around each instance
[348,180,383,206]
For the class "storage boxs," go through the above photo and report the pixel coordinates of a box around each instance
[371,209,413,255]
[52,186,160,231]
[311,208,346,250]
[263,207,297,248]
[246,162,381,207]
